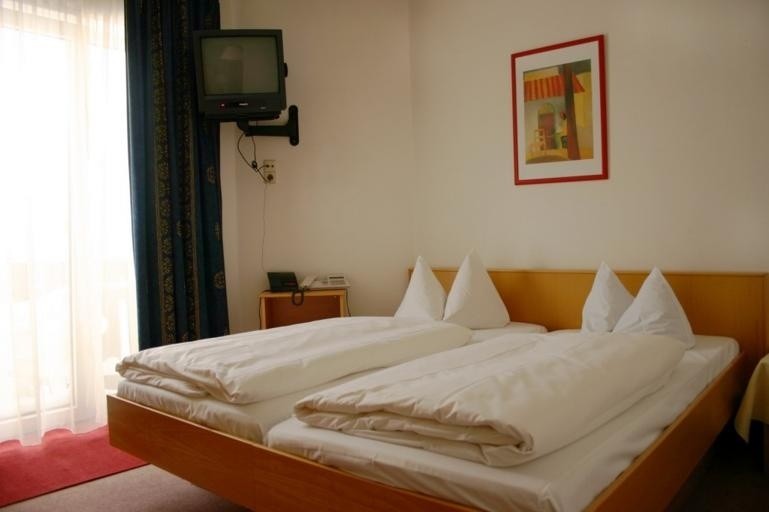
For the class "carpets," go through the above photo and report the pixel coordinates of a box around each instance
[0,424,151,507]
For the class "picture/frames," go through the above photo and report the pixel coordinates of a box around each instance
[511,33,609,185]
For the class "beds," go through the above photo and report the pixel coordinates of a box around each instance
[106,268,769,512]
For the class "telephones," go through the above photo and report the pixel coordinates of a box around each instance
[298,275,317,291]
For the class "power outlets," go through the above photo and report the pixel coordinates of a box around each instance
[263,160,277,184]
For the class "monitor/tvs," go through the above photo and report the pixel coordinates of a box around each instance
[187,29,288,121]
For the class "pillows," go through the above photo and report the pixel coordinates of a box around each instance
[580,263,696,347]
[396,255,512,330]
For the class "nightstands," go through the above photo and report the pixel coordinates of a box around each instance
[261,291,346,329]
